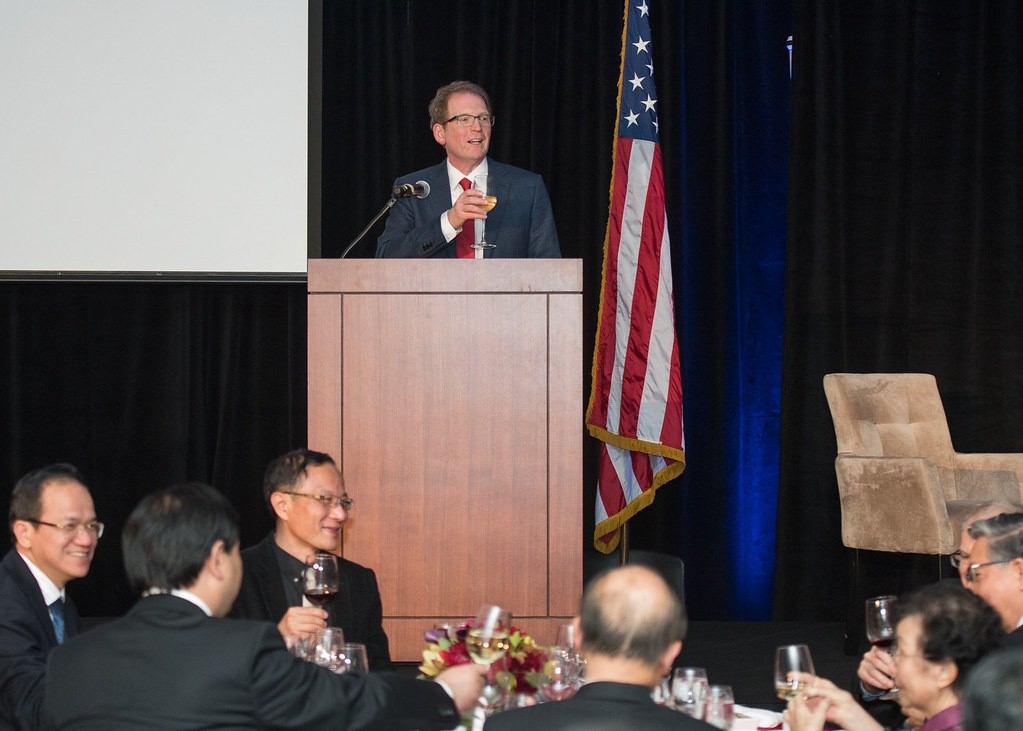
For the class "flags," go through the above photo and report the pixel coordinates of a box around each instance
[582,0,689,554]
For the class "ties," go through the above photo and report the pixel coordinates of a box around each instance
[50,596,67,646]
[455,177,476,259]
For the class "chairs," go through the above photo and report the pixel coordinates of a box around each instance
[824,371,1023,650]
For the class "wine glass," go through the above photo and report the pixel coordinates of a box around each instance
[866,596,900,700]
[470,175,497,249]
[470,656,511,718]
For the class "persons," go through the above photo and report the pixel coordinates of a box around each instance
[781,580,1009,731]
[0,460,104,730]
[223,447,396,674]
[485,563,724,731]
[838,498,1023,701]
[967,510,1023,646]
[374,75,562,259]
[40,480,491,731]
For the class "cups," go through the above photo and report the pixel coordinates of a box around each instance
[774,644,816,701]
[304,554,338,607]
[537,624,587,704]
[465,604,512,664]
[649,667,734,731]
[281,627,370,674]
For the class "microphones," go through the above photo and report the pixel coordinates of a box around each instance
[393,180,430,199]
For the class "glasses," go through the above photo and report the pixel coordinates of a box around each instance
[949,549,971,568]
[283,490,356,512]
[27,517,105,541]
[442,113,496,128]
[889,644,919,666]
[965,559,1012,584]
